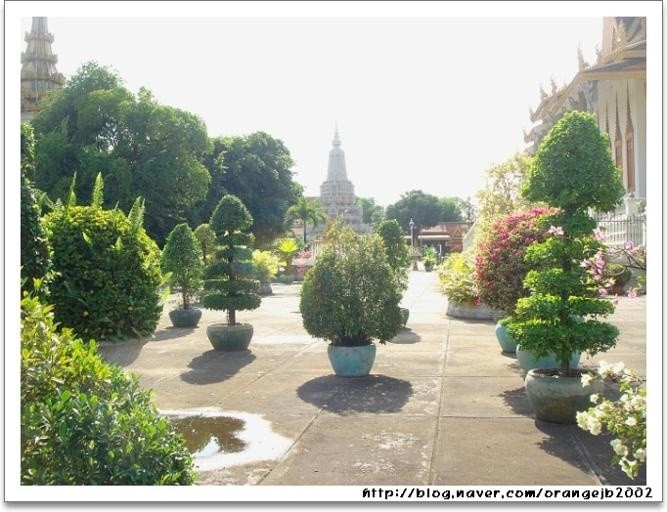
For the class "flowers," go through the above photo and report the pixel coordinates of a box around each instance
[573,358,645,480]
[472,203,556,320]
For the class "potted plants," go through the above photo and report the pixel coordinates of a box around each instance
[199,190,254,351]
[157,223,202,328]
[421,244,437,272]
[378,218,409,325]
[510,108,625,423]
[438,248,509,320]
[275,237,302,285]
[299,232,406,380]
[251,247,276,296]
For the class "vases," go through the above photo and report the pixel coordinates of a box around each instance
[514,339,581,372]
[493,319,519,353]
